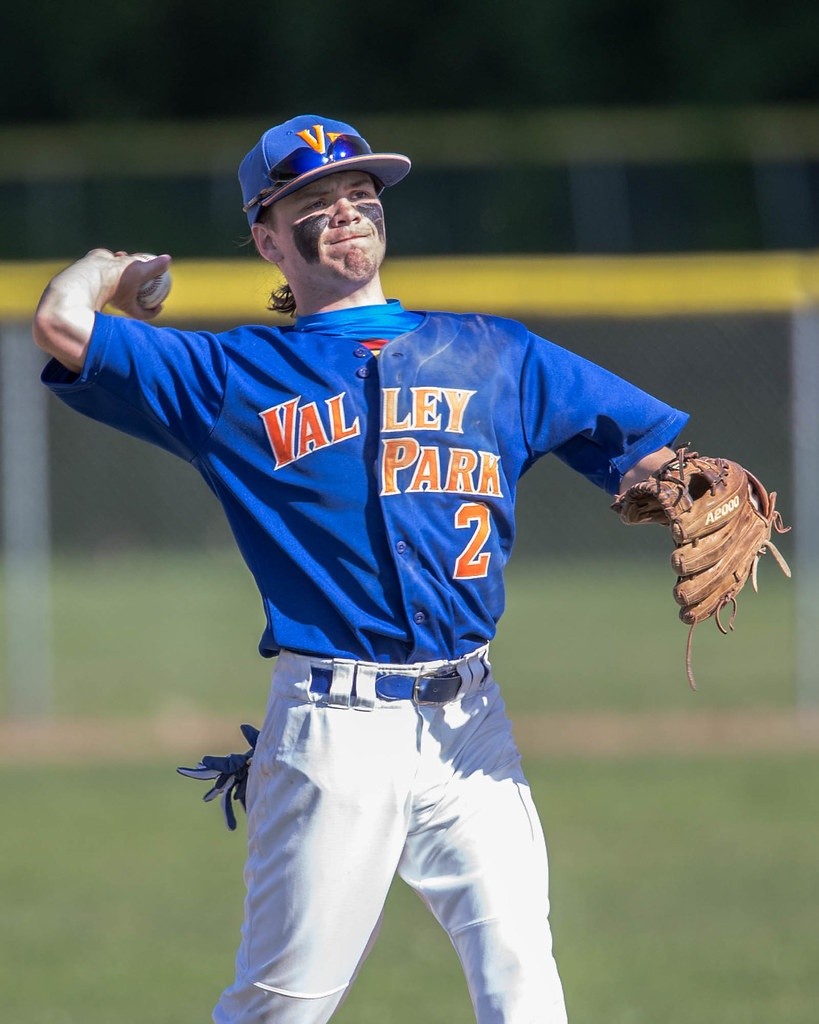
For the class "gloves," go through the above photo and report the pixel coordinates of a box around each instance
[176,724,260,830]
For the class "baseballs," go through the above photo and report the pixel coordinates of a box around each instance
[128,251,173,310]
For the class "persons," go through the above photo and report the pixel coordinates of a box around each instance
[32,116,793,1024]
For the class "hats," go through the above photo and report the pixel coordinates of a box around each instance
[237,115,412,228]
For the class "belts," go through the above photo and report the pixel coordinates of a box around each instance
[309,659,488,701]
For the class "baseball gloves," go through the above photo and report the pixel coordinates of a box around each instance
[608,440,779,627]
